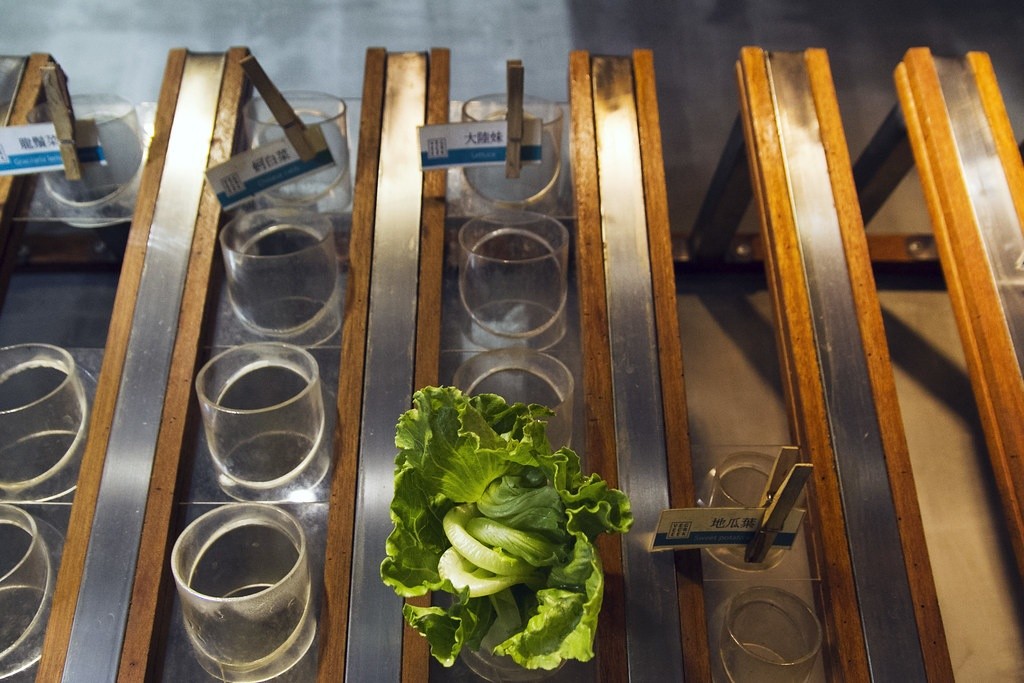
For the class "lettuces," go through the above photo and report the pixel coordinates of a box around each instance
[377,383,633,670]
